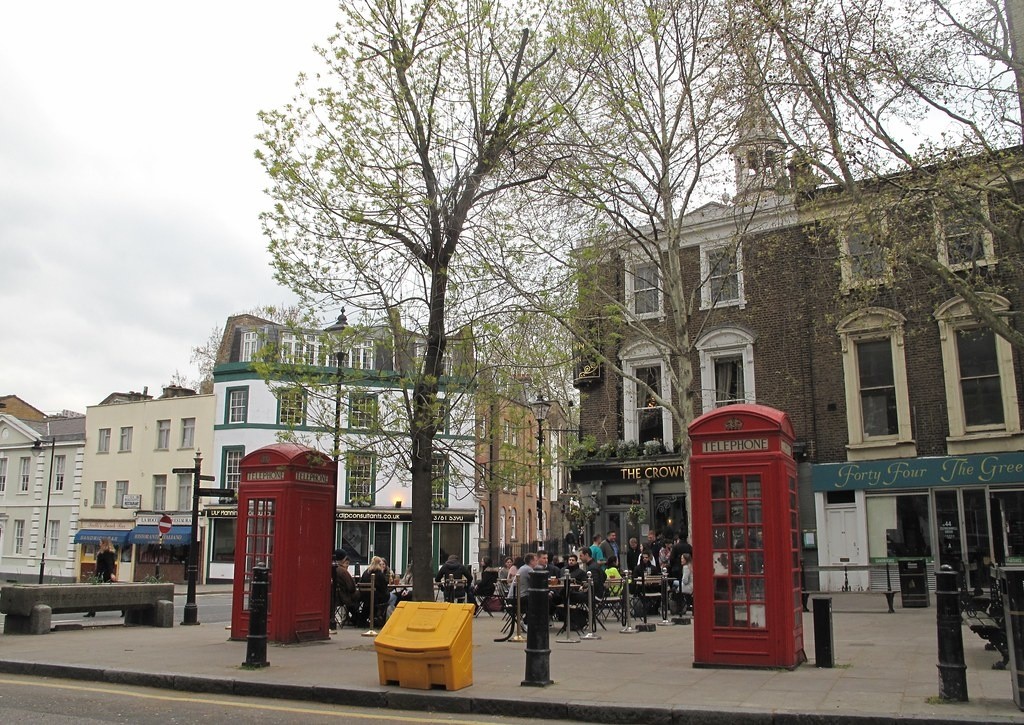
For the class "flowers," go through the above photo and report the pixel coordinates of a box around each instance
[572,438,664,461]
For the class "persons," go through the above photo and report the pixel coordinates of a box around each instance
[467,556,498,615]
[339,549,415,628]
[434,555,473,603]
[715,529,810,614]
[83,538,126,617]
[497,531,693,632]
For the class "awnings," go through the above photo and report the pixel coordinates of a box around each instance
[129,525,191,545]
[73,529,132,544]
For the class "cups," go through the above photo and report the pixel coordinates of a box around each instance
[610,573,615,580]
[551,576,556,586]
[394,575,400,585]
[449,574,453,581]
[644,571,647,578]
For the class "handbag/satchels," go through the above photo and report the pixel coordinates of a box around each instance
[484,597,504,613]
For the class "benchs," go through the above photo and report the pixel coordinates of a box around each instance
[962,603,1011,670]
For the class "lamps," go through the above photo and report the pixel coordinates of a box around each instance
[395,501,402,509]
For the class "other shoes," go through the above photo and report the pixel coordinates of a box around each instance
[83,611,95,618]
[120,610,125,617]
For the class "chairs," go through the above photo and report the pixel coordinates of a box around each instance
[333,563,682,643]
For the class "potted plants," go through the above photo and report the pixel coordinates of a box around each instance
[218,490,239,505]
[431,497,448,510]
[351,496,373,508]
[672,437,681,454]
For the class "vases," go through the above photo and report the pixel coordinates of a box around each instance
[637,445,665,457]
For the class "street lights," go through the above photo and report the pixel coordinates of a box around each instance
[323,305,359,559]
[529,388,551,559]
[30,435,55,584]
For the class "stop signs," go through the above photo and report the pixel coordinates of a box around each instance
[159,516,172,534]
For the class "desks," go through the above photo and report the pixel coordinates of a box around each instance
[635,575,679,617]
[547,584,581,635]
[387,584,413,608]
[595,579,625,622]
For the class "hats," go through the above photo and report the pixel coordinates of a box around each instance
[335,548,346,560]
